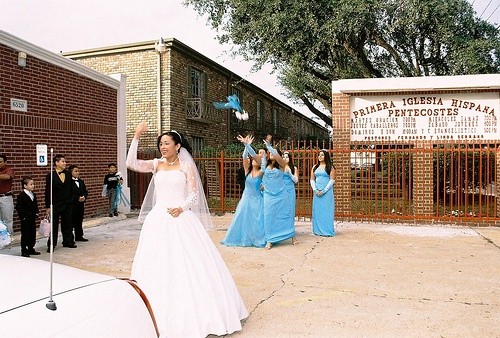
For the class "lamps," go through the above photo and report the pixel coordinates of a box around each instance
[158,38,166,54]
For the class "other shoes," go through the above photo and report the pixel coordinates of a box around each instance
[22,253,30,257]
[109,214,113,217]
[29,252,41,255]
[292,240,299,246]
[47,247,54,253]
[114,213,118,216]
[75,238,88,242]
[63,244,77,248]
[265,243,272,250]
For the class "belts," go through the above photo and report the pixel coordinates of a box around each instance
[0,192,12,197]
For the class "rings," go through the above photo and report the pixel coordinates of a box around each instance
[173,214,175,215]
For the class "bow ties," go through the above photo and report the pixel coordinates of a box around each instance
[58,170,65,175]
[74,180,79,183]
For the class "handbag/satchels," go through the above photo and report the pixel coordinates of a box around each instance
[39,214,51,237]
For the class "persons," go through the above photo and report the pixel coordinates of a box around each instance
[310,149,335,237]
[102,164,124,217]
[0,155,15,234]
[45,154,77,252]
[222,135,299,250]
[16,177,41,257]
[69,165,88,242]
[124,123,249,338]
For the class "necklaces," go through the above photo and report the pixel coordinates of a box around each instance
[167,157,179,165]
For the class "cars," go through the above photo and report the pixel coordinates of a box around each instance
[0,253,160,338]
[352,162,374,171]
